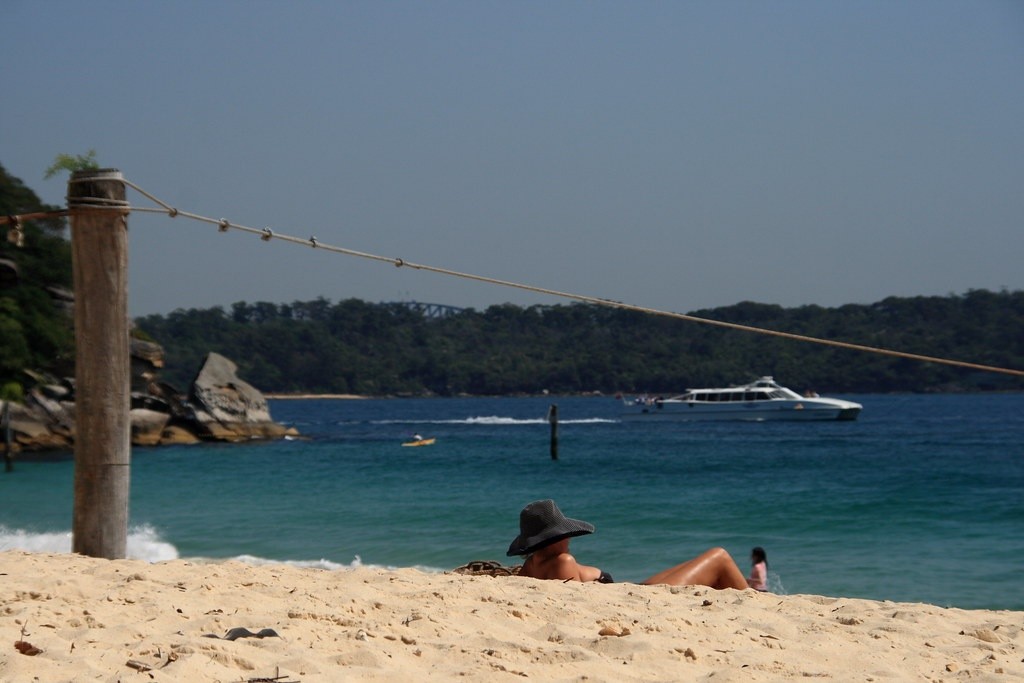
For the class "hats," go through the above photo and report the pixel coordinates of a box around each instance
[506,499,595,557]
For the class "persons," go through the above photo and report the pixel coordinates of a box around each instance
[745,547,768,592]
[805,388,820,399]
[413,432,423,441]
[506,500,750,592]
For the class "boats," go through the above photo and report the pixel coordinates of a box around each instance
[618,375,864,424]
